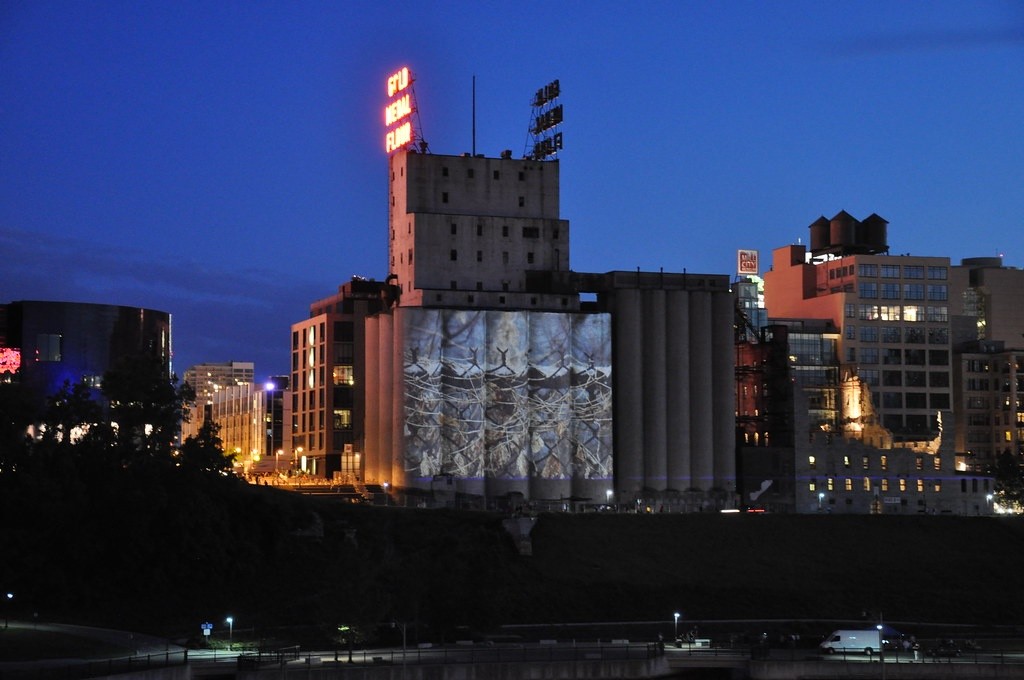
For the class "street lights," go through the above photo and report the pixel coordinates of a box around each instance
[819,492,825,510]
[384,483,389,505]
[606,489,613,505]
[226,617,234,647]
[673,613,681,641]
[294,447,303,473]
[237,381,251,457]
[267,383,275,458]
[987,495,994,509]
[275,449,283,474]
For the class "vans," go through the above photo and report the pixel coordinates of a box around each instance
[818,629,884,655]
